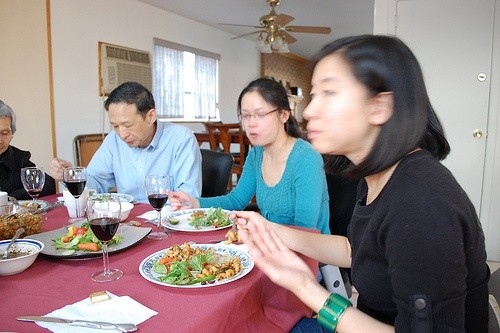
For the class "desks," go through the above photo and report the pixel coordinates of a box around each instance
[195,133,252,184]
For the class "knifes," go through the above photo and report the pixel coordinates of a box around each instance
[16,316,137,333]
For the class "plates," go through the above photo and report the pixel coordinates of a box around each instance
[161,208,234,232]
[23,218,152,261]
[10,200,49,213]
[138,244,255,288]
[88,193,134,202]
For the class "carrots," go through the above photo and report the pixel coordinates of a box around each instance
[77,243,98,251]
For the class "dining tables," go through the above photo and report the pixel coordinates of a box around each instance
[0,194,321,333]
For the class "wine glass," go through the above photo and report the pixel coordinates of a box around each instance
[145,175,171,240]
[87,193,124,282]
[63,166,87,224]
[21,167,45,210]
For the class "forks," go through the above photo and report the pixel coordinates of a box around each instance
[2,228,25,259]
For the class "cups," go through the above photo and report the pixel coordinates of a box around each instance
[63,187,87,218]
[0,192,8,206]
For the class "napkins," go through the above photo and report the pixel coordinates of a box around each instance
[136,205,174,221]
[34,291,159,333]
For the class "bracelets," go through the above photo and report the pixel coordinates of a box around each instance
[316,293,353,332]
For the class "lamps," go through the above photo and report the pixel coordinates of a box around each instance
[257,24,294,53]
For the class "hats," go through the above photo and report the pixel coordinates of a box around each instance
[238,108,279,121]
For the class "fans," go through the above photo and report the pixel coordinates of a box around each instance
[217,0,331,53]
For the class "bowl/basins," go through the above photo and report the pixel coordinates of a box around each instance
[0,205,47,239]
[93,202,134,222]
[0,239,44,276]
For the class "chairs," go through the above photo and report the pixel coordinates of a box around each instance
[199,121,243,199]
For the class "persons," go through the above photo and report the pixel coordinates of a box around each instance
[171,78,331,284]
[227,35,490,333]
[0,100,57,200]
[46,82,203,207]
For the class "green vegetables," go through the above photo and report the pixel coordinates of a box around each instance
[52,223,120,253]
[151,253,215,285]
[189,206,231,228]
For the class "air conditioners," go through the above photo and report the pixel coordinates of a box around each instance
[101,44,153,94]
